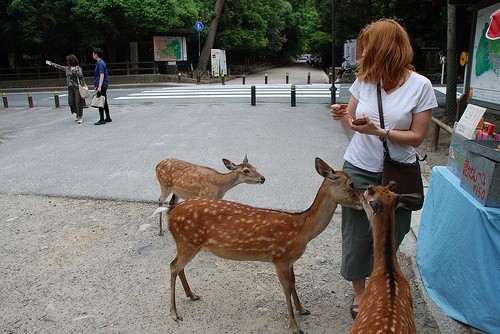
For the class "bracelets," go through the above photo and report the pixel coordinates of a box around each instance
[379,129,389,141]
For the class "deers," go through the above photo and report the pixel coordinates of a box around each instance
[155,155,425,334]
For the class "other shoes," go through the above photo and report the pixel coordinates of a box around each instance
[350,295,359,321]
[75,114,78,120]
[105,118,111,122]
[77,118,84,123]
[95,119,106,125]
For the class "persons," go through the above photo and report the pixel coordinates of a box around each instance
[45,54,86,124]
[335,58,347,83]
[330,17,438,321]
[306,54,320,68]
[93,48,112,125]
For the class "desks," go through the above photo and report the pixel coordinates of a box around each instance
[415,166,500,334]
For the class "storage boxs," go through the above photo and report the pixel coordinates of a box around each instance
[460,150,499,207]
[447,130,471,179]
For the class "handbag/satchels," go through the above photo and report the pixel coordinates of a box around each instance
[78,84,90,99]
[381,153,424,211]
[91,92,105,109]
